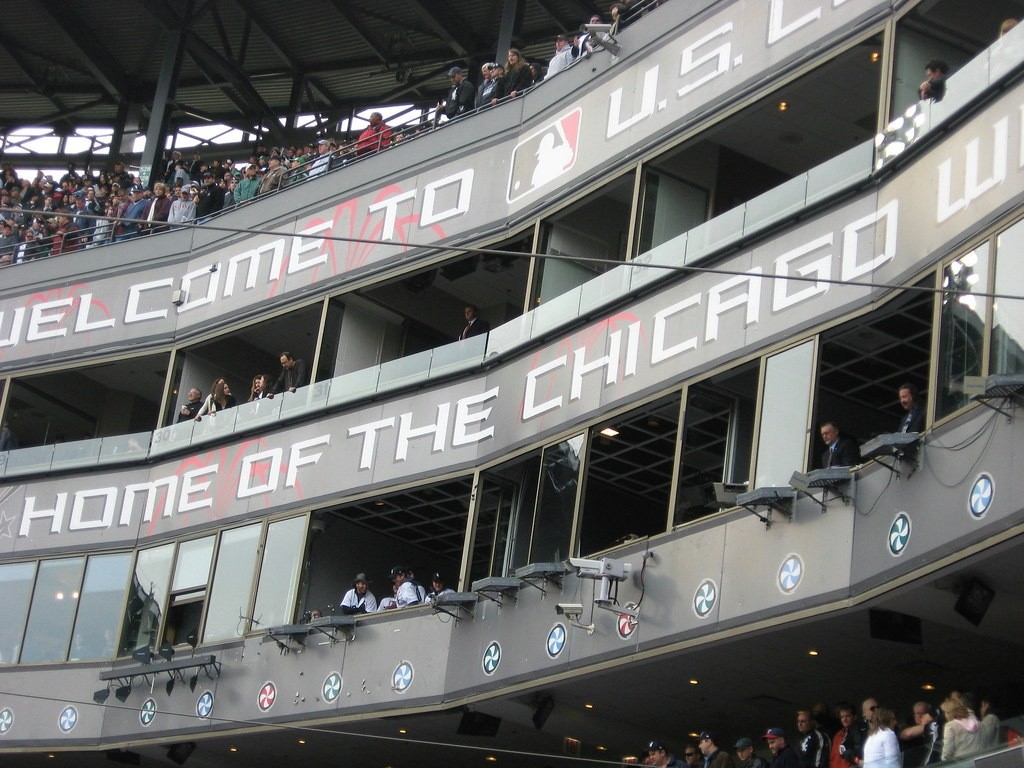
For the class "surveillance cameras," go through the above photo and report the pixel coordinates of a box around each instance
[555,603,583,616]
[579,24,611,34]
[564,558,632,581]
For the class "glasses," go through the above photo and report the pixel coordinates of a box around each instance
[683,753,693,756]
[490,68,495,72]
[871,706,881,711]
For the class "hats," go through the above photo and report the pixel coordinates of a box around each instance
[202,172,214,177]
[695,731,712,740]
[235,173,241,176]
[763,728,783,738]
[388,567,404,578]
[351,573,372,584]
[733,738,752,747]
[554,35,568,41]
[269,155,281,162]
[432,571,442,582]
[113,183,120,188]
[447,67,461,77]
[313,140,329,146]
[226,158,234,164]
[132,185,143,191]
[644,739,664,750]
[33,218,46,223]
[488,63,503,69]
[182,186,189,194]
[246,164,256,170]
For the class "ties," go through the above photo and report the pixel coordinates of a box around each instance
[826,449,832,467]
[465,323,471,332]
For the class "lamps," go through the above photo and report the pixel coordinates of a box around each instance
[105,749,140,766]
[113,677,132,702]
[165,669,177,696]
[159,641,177,660]
[311,616,356,645]
[518,562,566,594]
[601,423,620,438]
[166,742,196,766]
[186,629,201,657]
[971,372,1024,423]
[434,593,480,619]
[530,694,558,729]
[867,611,927,644]
[132,644,153,664]
[472,577,520,610]
[262,625,310,654]
[736,487,795,527]
[190,664,202,692]
[791,468,852,513]
[456,709,501,737]
[860,432,920,479]
[954,576,995,624]
[93,681,111,704]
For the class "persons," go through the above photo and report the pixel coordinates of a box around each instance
[818,421,861,471]
[918,59,957,102]
[311,610,323,619]
[1000,17,1018,38]
[898,383,925,434]
[340,568,457,616]
[459,303,490,342]
[0,111,432,267]
[635,691,1000,768]
[438,49,543,120]
[177,351,310,423]
[543,0,655,81]
